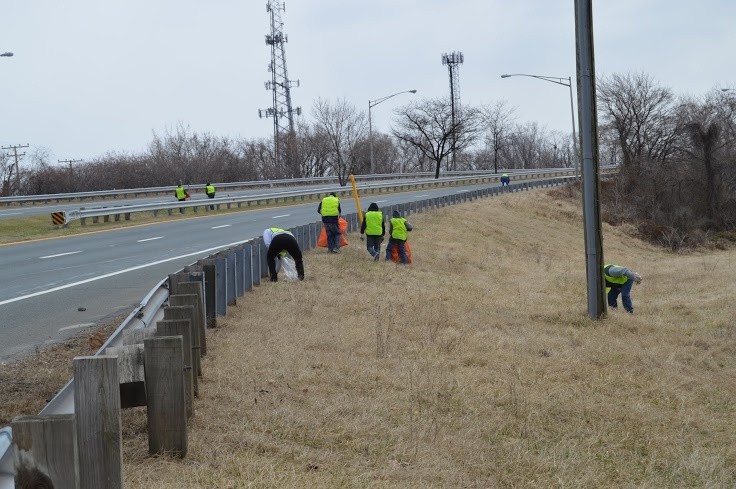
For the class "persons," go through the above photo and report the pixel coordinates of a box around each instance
[205,182,215,211]
[263,226,305,281]
[385,212,414,264]
[604,264,642,314]
[359,204,386,260]
[175,184,188,214]
[500,173,510,186]
[318,191,341,254]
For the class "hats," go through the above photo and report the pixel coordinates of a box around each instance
[368,202,379,211]
[393,211,401,218]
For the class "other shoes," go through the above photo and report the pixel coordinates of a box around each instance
[328,249,341,253]
[374,253,379,261]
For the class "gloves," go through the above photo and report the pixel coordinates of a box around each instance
[360,234,364,242]
[381,236,384,242]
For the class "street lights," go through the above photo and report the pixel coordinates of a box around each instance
[501,73,579,185]
[368,90,418,173]
